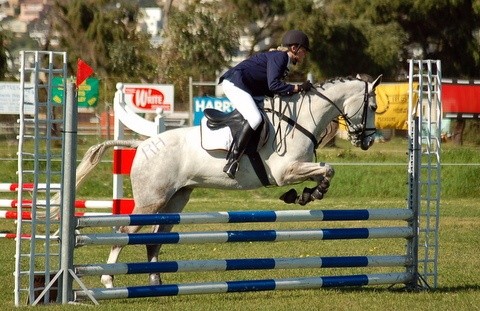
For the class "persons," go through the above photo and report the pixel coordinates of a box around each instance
[218,30,314,179]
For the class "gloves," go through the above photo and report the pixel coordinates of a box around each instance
[297,79,312,93]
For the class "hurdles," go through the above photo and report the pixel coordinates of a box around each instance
[30,82,435,310]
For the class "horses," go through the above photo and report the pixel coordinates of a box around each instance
[36,73,383,290]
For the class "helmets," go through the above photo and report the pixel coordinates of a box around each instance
[282,29,312,53]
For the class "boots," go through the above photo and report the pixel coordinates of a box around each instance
[223,120,256,179]
